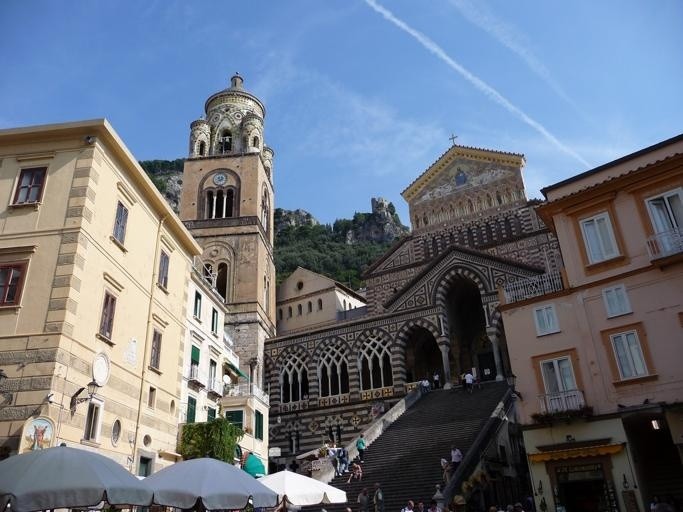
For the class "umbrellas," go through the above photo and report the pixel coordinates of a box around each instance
[254,467,347,512]
[0,442,154,511]
[139,454,281,512]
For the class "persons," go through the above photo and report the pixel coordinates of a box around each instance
[355,486,369,512]
[414,500,428,511]
[513,502,523,511]
[649,493,658,511]
[401,500,414,512]
[452,495,467,512]
[427,499,442,511]
[507,504,514,510]
[490,506,497,510]
[655,495,674,511]
[449,443,462,471]
[372,482,384,512]
[526,497,533,511]
[355,434,366,463]
[457,368,481,393]
[344,462,363,485]
[421,376,431,394]
[440,457,454,486]
[327,442,343,478]
[335,444,350,476]
[432,371,440,389]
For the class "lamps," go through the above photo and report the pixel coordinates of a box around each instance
[71,379,99,411]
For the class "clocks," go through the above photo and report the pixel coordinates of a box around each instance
[213,172,228,186]
